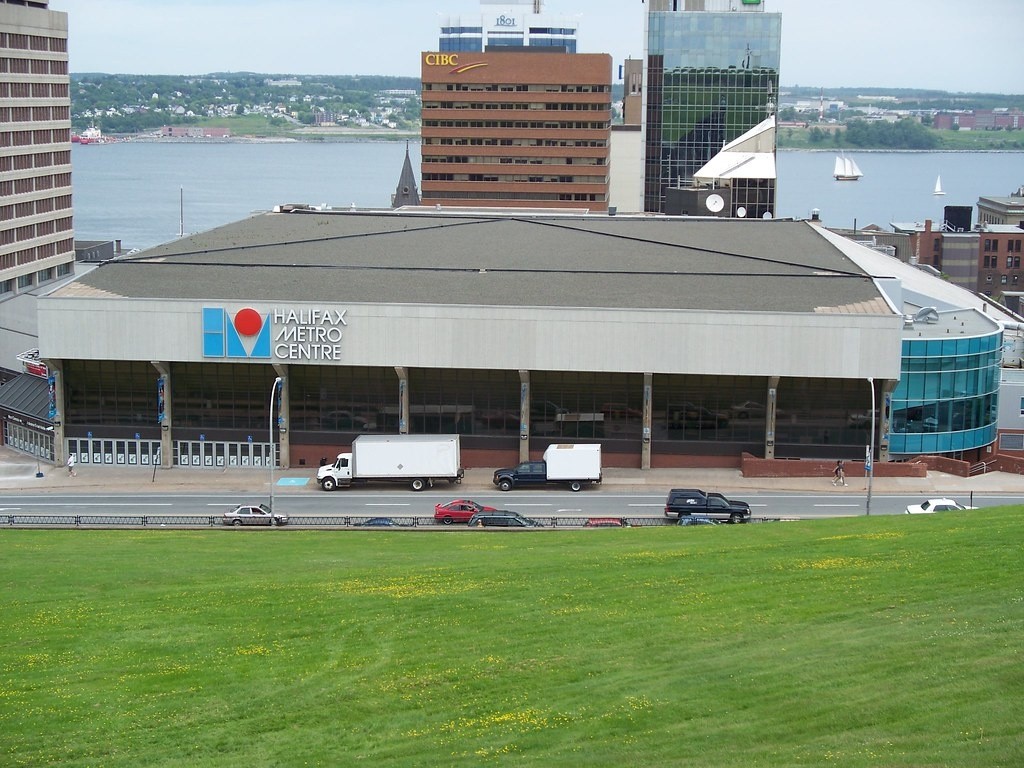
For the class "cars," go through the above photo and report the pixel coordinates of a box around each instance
[433,499,497,525]
[582,518,630,528]
[483,411,533,429]
[222,504,291,526]
[353,517,403,527]
[906,498,978,514]
[732,402,783,421]
[676,516,722,525]
[601,403,642,417]
[320,412,368,430]
[851,408,880,428]
[532,400,571,414]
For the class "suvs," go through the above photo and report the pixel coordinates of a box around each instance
[668,402,731,429]
[467,510,545,528]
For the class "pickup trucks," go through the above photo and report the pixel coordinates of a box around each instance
[664,488,752,524]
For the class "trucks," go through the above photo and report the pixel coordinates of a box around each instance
[316,435,465,492]
[365,405,476,436]
[494,443,604,492]
[534,413,606,439]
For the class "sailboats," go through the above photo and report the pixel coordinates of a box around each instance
[933,175,946,196]
[833,155,863,182]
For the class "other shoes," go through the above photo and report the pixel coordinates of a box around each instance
[831,479,848,487]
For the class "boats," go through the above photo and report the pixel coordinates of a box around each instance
[71,128,105,145]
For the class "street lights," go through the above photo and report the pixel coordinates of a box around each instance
[866,377,876,513]
[269,377,282,528]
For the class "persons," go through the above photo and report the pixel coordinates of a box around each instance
[67,454,76,477]
[832,459,848,487]
